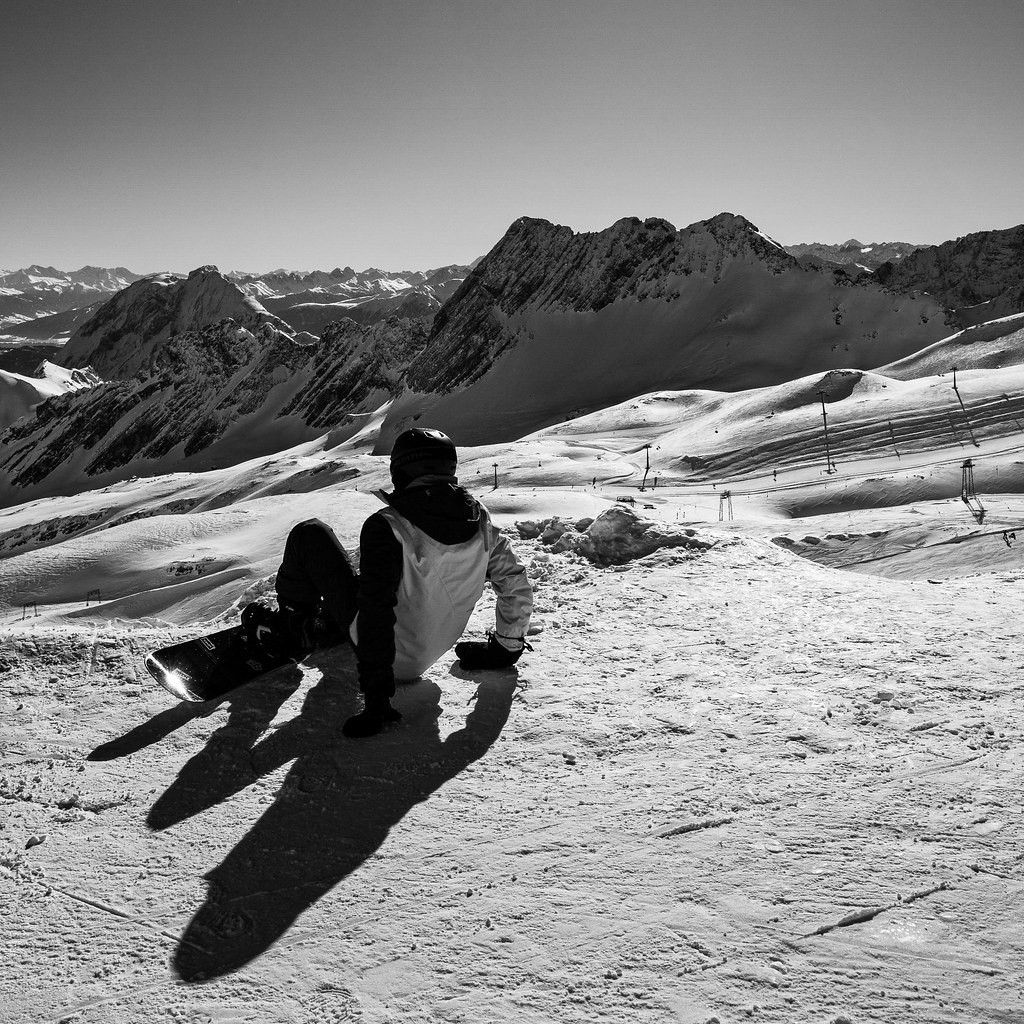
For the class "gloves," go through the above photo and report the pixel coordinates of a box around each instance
[455,633,526,671]
[342,696,403,740]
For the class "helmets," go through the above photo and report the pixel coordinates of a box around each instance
[390,427,458,489]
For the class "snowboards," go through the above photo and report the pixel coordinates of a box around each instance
[141,607,355,705]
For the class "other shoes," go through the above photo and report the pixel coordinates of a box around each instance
[241,602,312,664]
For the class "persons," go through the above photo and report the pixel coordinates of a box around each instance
[239,427,534,739]
